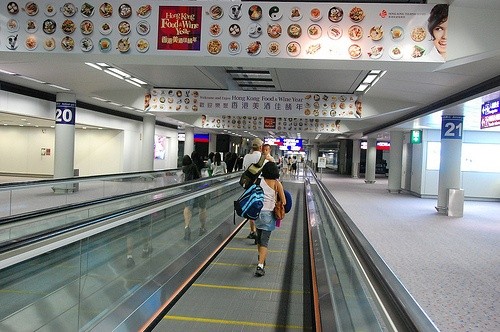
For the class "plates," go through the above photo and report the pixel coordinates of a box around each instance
[206,115,263,130]
[277,118,335,132]
[304,94,354,117]
[207,4,262,56]
[151,90,199,112]
[6,2,57,52]
[267,6,323,57]
[117,3,152,53]
[328,6,365,59]
[367,26,426,60]
[61,3,113,52]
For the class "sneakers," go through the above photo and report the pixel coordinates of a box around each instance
[255,255,266,276]
[126,257,136,266]
[142,245,153,258]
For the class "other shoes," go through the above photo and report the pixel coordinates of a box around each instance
[199,227,207,236]
[247,231,258,244]
[184,225,191,240]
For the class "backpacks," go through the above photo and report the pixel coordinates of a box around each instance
[233,178,264,224]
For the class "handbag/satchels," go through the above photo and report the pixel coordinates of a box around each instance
[274,200,285,220]
[239,163,263,189]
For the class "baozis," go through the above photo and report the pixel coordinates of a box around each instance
[8,3,18,12]
[230,25,240,34]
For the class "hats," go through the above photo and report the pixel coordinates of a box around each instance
[251,137,264,148]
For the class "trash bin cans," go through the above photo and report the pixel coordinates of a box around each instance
[73,169,80,192]
[448,189,464,216]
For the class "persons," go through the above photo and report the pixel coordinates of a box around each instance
[181,151,297,240]
[262,145,275,163]
[242,137,270,239]
[145,92,151,111]
[201,114,206,128]
[126,213,155,263]
[353,96,361,119]
[252,161,287,276]
[427,4,449,58]
[335,120,340,132]
